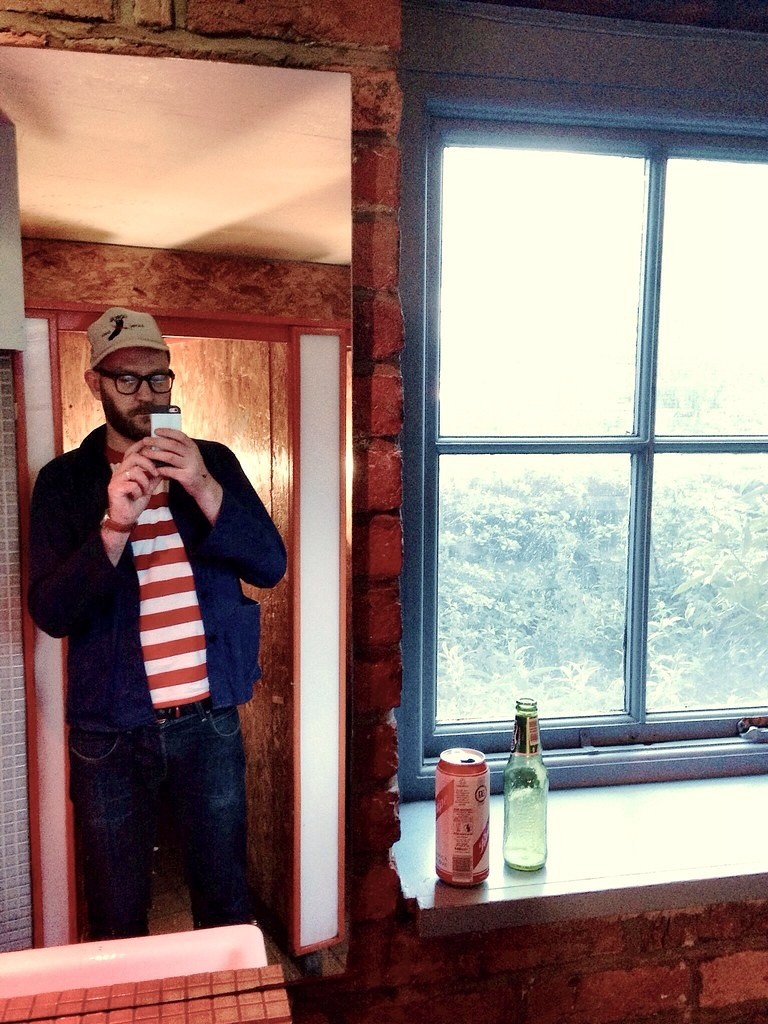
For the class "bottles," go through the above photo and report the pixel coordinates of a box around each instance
[503,697,548,871]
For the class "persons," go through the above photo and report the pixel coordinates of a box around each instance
[26,307,288,943]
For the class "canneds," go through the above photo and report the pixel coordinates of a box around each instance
[435,748,490,886]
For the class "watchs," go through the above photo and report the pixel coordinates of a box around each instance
[100,508,137,532]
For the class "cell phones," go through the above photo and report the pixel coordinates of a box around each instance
[149,405,182,468]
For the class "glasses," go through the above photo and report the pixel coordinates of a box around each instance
[93,366,175,395]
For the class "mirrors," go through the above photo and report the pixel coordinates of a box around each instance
[0,45,352,979]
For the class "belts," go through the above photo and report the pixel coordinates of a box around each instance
[156,696,213,722]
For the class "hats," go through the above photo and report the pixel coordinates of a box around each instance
[86,307,169,369]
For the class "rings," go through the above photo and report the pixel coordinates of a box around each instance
[126,470,131,482]
